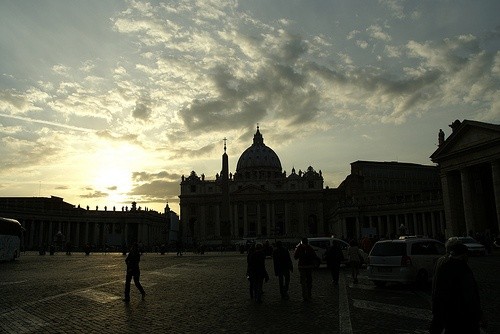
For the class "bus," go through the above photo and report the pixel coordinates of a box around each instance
[0,217,26,263]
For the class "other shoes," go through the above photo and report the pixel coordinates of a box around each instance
[304,298,310,304]
[122,297,131,303]
[141,291,145,300]
[282,294,289,300]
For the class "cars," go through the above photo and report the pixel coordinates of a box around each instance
[367,239,448,294]
[445,237,485,259]
[294,238,369,266]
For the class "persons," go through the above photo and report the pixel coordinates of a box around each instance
[247,239,361,302]
[37,233,404,257]
[429,229,494,334]
[121,242,146,302]
